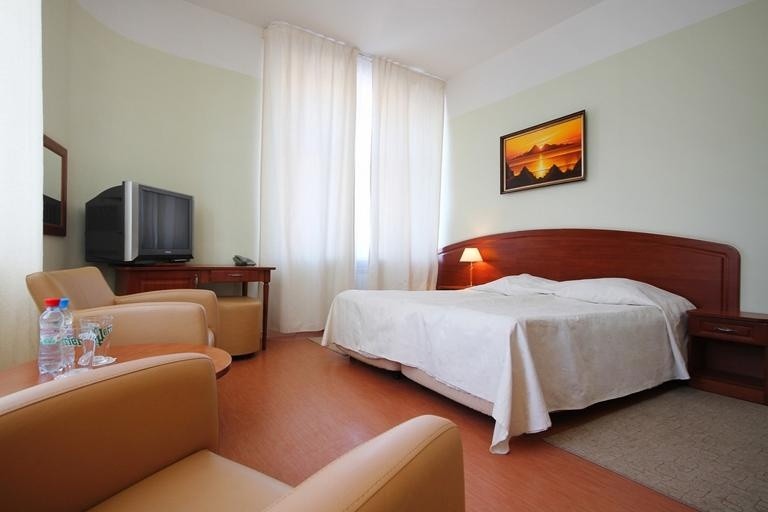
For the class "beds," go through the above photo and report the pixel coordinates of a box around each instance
[319,227,740,456]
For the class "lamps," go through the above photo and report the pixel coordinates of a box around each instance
[458,245,485,287]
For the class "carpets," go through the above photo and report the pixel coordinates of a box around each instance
[539,383,768,512]
[304,334,349,357]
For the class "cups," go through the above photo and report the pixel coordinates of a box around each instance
[79,314,114,365]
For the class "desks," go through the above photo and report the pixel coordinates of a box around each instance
[1,343,233,399]
[114,264,276,351]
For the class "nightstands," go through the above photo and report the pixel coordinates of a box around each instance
[685,307,768,407]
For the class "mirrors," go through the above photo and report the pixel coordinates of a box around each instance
[42,133,68,238]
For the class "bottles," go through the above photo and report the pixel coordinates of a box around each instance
[58,297,79,369]
[35,298,65,378]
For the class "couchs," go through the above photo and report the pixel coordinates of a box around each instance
[215,295,263,357]
[1,351,468,512]
[23,265,221,349]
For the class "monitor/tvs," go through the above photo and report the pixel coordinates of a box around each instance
[85,180,193,266]
[43,193,62,230]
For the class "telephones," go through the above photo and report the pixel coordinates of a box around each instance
[233,255,256,266]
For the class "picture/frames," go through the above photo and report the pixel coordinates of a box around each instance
[499,108,587,195]
